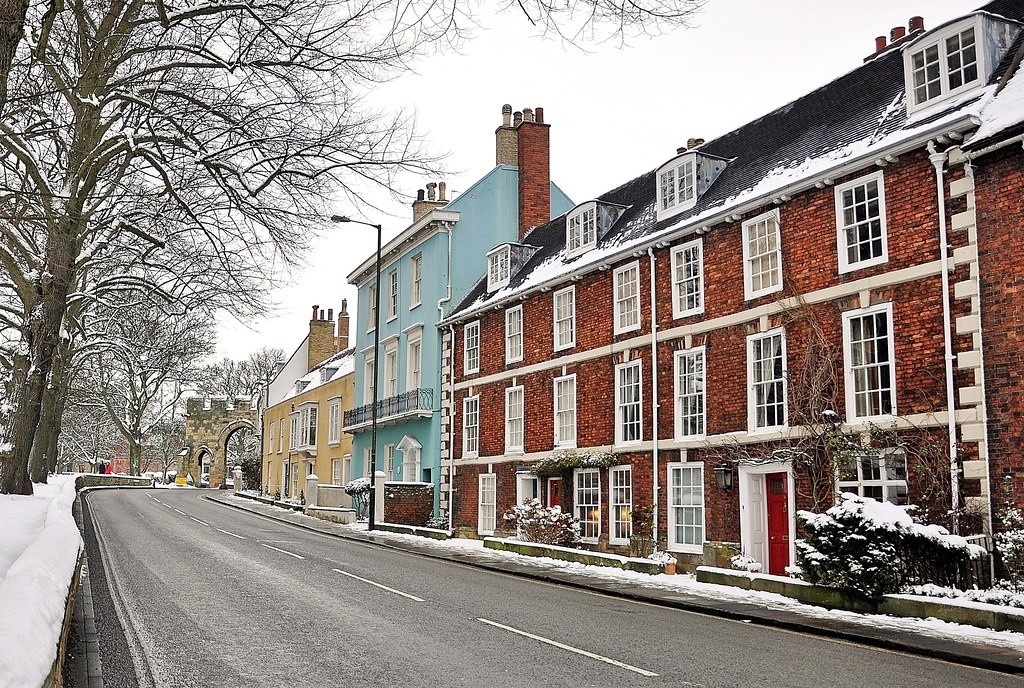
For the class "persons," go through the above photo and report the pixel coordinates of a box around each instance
[168,475,171,484]
[99,461,111,474]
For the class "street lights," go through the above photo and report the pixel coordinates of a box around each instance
[329,213,383,532]
